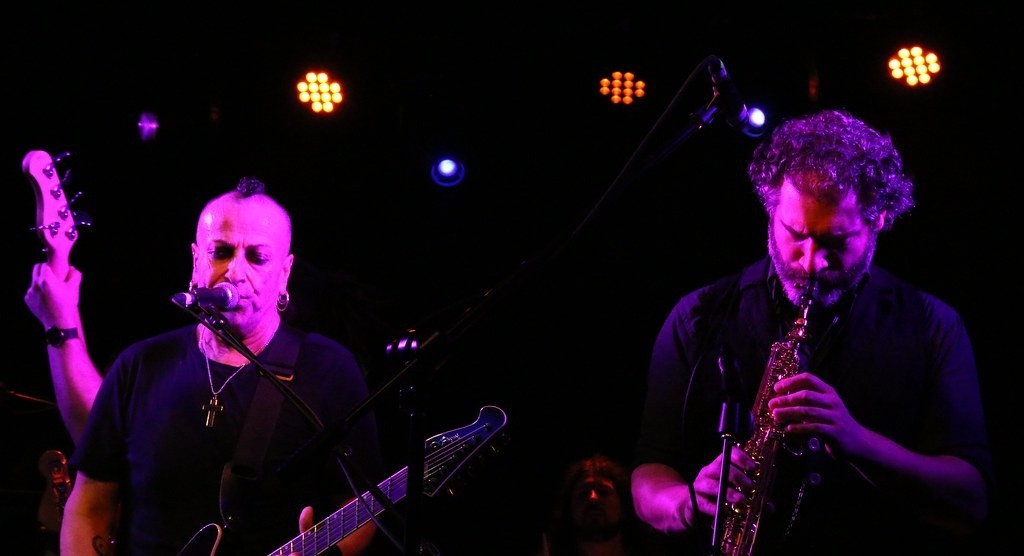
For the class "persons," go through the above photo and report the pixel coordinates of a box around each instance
[541,110,988,556]
[25,177,381,556]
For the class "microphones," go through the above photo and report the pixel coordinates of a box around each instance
[174,281,241,311]
[708,58,750,129]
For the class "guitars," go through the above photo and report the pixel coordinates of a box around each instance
[177,400,514,556]
[21,146,96,358]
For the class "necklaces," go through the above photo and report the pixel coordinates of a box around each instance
[197,328,273,428]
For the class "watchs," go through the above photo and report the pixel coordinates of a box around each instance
[46,327,78,348]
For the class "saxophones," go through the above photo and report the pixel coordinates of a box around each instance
[707,278,824,556]
